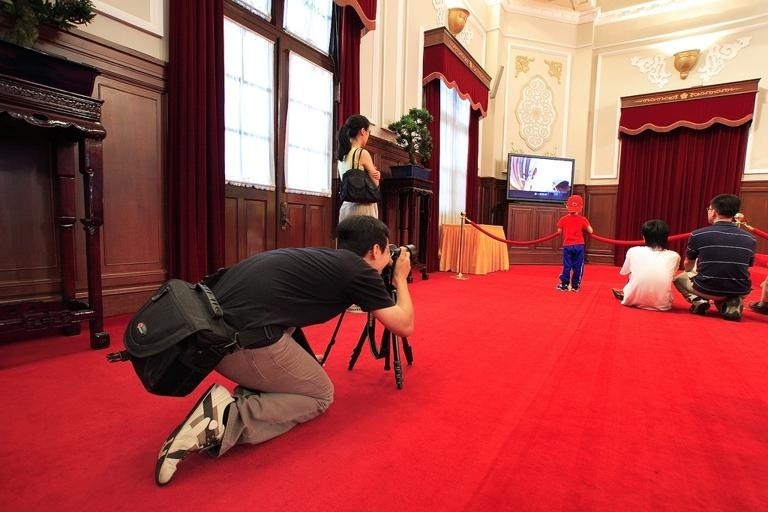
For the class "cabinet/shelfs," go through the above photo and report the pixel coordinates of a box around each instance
[507,204,568,265]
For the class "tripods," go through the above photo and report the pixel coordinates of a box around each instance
[348,272,414,390]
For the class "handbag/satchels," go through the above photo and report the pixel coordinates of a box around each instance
[339,167,383,203]
[106,278,234,397]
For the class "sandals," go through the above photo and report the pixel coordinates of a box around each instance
[749,300,768,315]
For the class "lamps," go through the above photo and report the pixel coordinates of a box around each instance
[448,8,469,37]
[674,49,701,80]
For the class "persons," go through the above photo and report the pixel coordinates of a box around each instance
[612,218,681,312]
[335,113,383,314]
[672,194,757,321]
[749,275,768,315]
[155,215,418,486]
[552,180,572,198]
[556,194,593,293]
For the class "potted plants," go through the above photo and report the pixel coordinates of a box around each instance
[0,0,99,95]
[389,109,434,178]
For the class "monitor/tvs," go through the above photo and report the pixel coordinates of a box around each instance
[507,152,575,207]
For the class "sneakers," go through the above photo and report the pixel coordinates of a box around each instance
[154,383,237,487]
[611,288,624,300]
[689,297,711,314]
[572,283,581,292]
[557,282,570,291]
[721,295,743,321]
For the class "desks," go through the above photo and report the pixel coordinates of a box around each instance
[382,178,433,282]
[438,224,510,275]
[1,83,108,348]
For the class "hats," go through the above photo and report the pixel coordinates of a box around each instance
[566,195,584,213]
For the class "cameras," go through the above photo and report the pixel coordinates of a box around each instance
[387,244,418,267]
